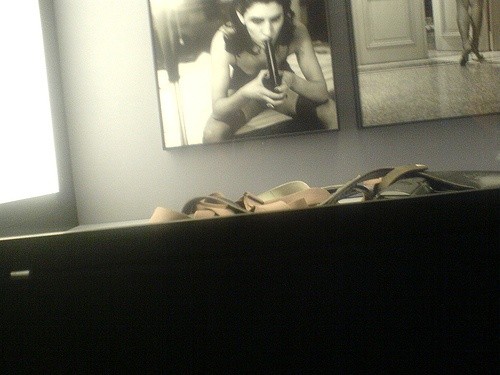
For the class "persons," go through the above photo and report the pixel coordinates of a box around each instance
[203,0,338,144]
[456,0,484,67]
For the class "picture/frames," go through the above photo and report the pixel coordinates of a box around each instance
[148,1,341,150]
[345,1,499,128]
